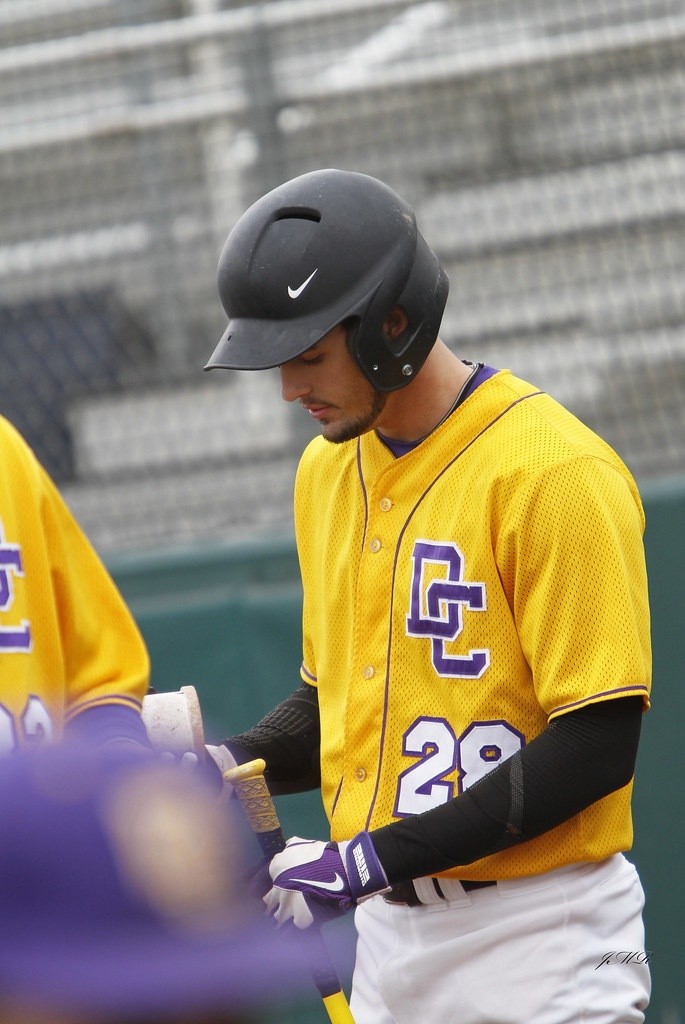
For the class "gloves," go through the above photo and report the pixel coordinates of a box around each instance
[258,831,391,930]
[164,732,252,801]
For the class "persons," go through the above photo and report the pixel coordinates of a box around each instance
[1,412,151,785]
[160,169,651,1023]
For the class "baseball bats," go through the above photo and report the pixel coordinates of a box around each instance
[223,758,356,1024]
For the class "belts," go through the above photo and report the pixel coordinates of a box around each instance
[387,866,496,909]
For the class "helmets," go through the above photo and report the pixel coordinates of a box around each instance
[198,168,450,391]
